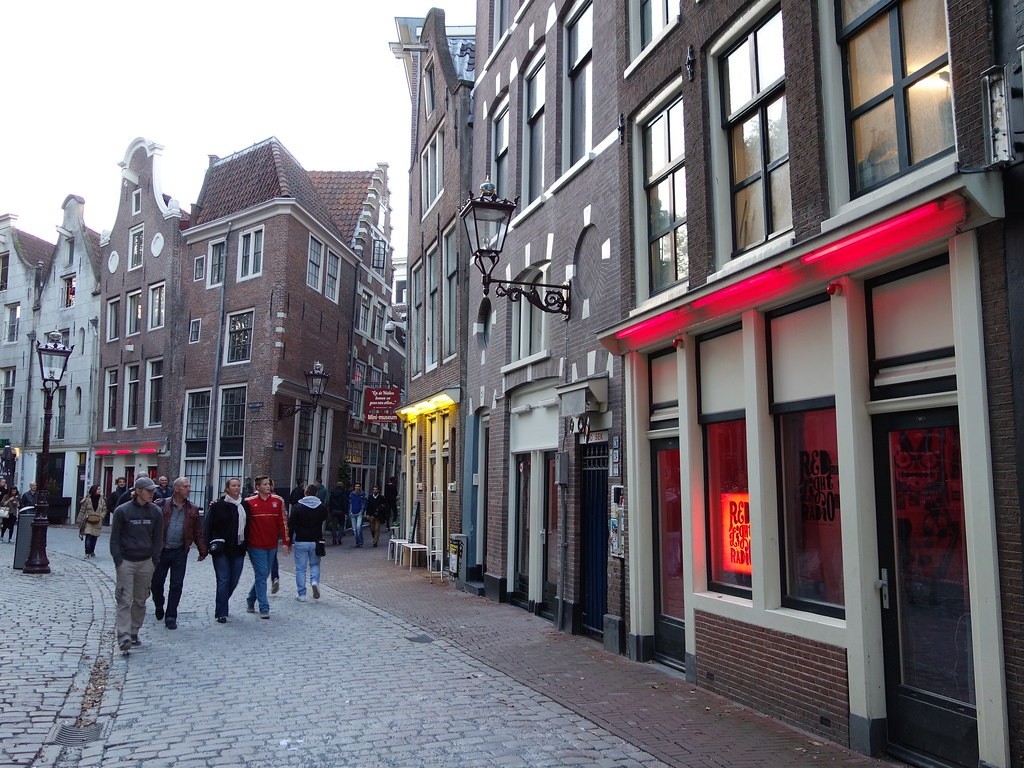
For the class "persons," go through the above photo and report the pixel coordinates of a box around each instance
[203,475,291,623]
[384,477,398,530]
[330,481,385,547]
[0,479,38,543]
[289,478,329,601]
[151,476,209,629]
[78,471,172,558]
[109,477,164,650]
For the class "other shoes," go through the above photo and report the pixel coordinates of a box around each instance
[260,612,270,619]
[91,553,95,557]
[8,539,15,544]
[373,544,377,548]
[358,544,363,548]
[338,539,342,545]
[311,582,320,599]
[165,618,177,629]
[0,536,3,541]
[385,527,390,531]
[218,616,227,623]
[84,554,89,559]
[246,598,255,613]
[332,539,337,545]
[121,640,131,651]
[131,634,141,645]
[155,607,164,620]
[296,595,306,602]
[271,579,280,595]
[354,544,359,548]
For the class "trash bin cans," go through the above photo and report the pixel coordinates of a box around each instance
[14,506,36,570]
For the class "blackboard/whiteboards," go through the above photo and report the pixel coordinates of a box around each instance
[408,500,419,544]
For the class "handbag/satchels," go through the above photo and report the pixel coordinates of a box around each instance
[208,539,225,557]
[316,538,326,556]
[87,514,100,523]
[0,504,10,519]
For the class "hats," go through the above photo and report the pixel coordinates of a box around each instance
[135,477,160,490]
[137,471,149,479]
[336,482,344,487]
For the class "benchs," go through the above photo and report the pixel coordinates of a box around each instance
[387,538,408,565]
[400,543,430,571]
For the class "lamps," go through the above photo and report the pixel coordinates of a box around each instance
[455,173,571,320]
[277,361,331,421]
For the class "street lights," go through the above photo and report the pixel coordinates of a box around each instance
[23,330,75,574]
